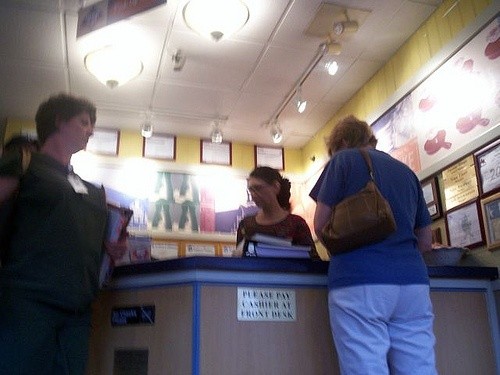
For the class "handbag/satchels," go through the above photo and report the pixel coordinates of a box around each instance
[319,147,396,255]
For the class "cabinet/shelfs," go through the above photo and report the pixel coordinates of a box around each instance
[114,227,238,268]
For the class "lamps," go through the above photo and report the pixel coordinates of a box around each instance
[290,84,307,113]
[182,0,251,44]
[319,59,338,76]
[317,33,343,57]
[332,8,360,36]
[269,118,285,144]
[167,47,189,73]
[83,43,144,93]
[140,113,154,139]
[209,119,226,145]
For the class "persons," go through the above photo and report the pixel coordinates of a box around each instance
[0,94,132,375]
[309,116,441,375]
[235,167,322,260]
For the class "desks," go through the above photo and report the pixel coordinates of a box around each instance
[84,254,500,375]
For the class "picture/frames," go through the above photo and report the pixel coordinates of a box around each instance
[200,137,233,167]
[253,143,286,171]
[479,192,500,252]
[83,126,121,157]
[475,141,500,198]
[419,173,443,223]
[142,132,177,162]
[440,152,482,213]
[442,196,487,250]
